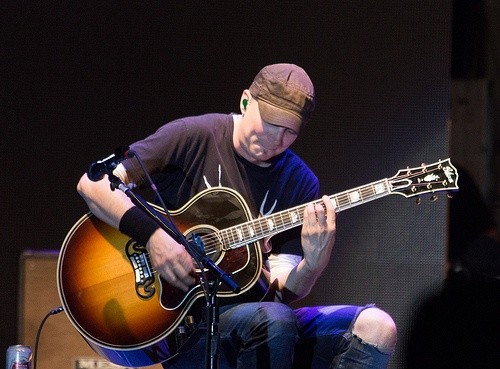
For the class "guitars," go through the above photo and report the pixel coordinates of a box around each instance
[56,156,460,368]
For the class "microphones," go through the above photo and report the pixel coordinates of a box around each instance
[87,149,134,181]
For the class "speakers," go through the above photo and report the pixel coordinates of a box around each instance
[18,251,164,369]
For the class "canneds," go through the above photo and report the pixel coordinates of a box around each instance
[6,344,32,369]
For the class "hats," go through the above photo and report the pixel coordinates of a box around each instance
[249,62,315,135]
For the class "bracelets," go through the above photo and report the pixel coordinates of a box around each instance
[119,206,163,247]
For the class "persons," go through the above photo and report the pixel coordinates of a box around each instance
[77,64,398,369]
